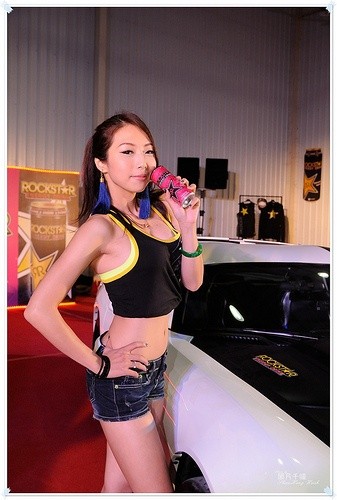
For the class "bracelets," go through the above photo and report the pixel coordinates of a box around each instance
[180,242,203,258]
[91,355,111,379]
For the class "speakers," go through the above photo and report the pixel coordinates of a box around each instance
[176,157,200,189]
[205,158,228,190]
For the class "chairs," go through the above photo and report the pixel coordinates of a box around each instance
[283,267,331,292]
[205,280,263,329]
[280,291,331,334]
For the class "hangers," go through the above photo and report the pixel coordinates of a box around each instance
[267,197,282,207]
[238,196,258,207]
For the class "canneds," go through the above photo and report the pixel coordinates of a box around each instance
[29,200,68,298]
[149,165,195,208]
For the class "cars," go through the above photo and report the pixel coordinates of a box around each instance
[94,236,330,493]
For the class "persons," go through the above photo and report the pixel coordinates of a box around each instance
[22,112,205,492]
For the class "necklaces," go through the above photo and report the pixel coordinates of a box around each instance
[124,212,152,230]
